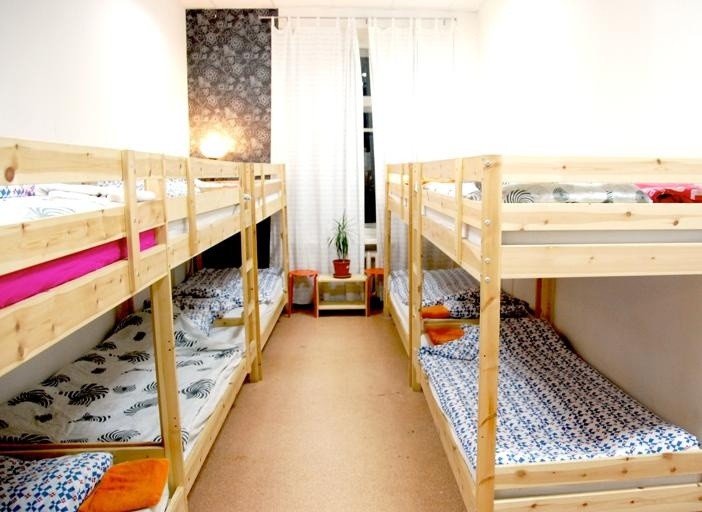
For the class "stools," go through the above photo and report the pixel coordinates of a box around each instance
[288,270,317,317]
[364,269,384,317]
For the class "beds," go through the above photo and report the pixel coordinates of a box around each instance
[0,136,289,512]
[382,154,702,512]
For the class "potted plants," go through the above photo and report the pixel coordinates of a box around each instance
[323,208,364,278]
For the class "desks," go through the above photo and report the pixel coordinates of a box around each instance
[317,274,368,318]
